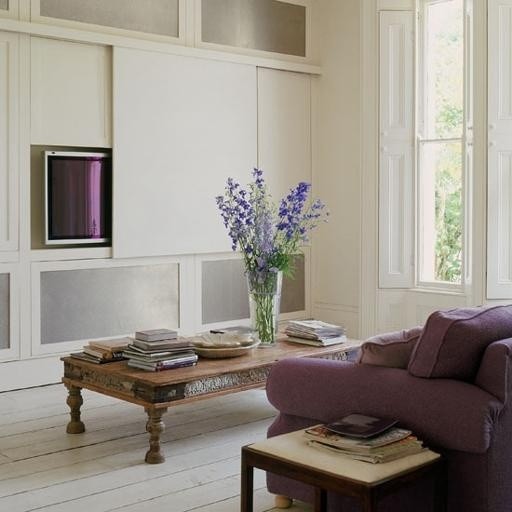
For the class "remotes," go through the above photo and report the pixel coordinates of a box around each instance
[210,329,224,334]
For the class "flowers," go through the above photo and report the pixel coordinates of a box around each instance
[216,166,331,276]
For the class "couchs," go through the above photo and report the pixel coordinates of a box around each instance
[263,335,509,511]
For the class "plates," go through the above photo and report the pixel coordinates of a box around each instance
[190,331,262,359]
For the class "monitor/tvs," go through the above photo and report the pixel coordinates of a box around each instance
[40,150,112,245]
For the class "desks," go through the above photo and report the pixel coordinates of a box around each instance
[238,425,449,510]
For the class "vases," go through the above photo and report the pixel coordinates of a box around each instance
[246,266,280,343]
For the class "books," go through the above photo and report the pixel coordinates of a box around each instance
[302,410,430,464]
[284,319,348,348]
[70,328,199,373]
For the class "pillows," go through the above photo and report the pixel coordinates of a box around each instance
[360,323,416,363]
[410,300,511,379]
